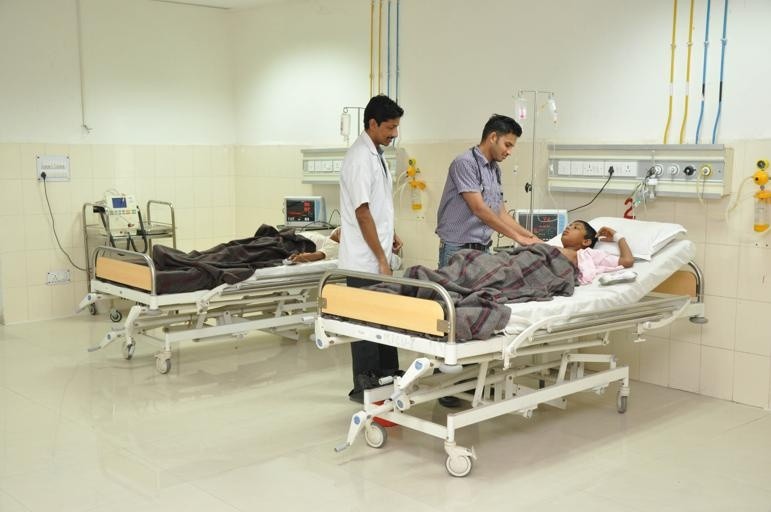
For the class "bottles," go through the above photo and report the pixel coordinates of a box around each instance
[411,186,423,210]
[515,98,530,130]
[547,98,560,124]
[753,199,770,232]
[340,113,352,137]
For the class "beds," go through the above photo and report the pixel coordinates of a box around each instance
[90,243,403,375]
[312,262,705,479]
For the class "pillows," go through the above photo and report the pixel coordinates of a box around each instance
[544,215,688,262]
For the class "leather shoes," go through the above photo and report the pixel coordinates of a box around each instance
[457,378,494,396]
[433,368,459,407]
[349,390,364,404]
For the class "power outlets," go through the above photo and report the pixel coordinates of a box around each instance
[583,161,638,178]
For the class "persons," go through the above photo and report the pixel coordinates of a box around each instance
[551,220,636,284]
[433,112,549,407]
[285,226,341,264]
[339,94,405,405]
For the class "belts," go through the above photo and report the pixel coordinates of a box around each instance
[458,240,493,251]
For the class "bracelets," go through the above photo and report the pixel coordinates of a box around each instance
[611,233,624,244]
[529,234,535,238]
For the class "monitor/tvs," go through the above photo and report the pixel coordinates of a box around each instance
[284,195,327,228]
[515,208,566,242]
